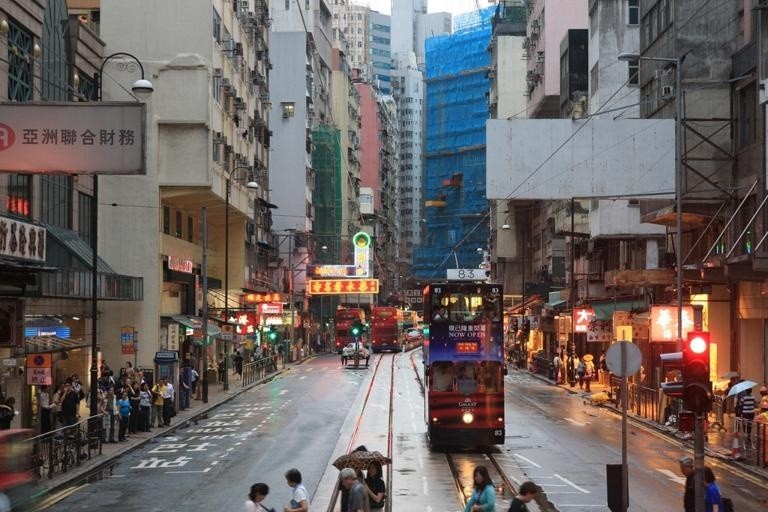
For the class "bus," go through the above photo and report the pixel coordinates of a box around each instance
[371,305,405,353]
[420,267,507,446]
[334,308,368,354]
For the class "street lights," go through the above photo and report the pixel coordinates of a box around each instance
[501,213,525,350]
[682,326,713,412]
[87,50,156,446]
[476,247,491,262]
[615,51,684,338]
[291,242,329,346]
[221,165,260,392]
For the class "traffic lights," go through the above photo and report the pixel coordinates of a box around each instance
[270,332,278,341]
[358,323,365,335]
[351,323,360,337]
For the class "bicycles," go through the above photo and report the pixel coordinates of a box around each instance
[45,434,78,476]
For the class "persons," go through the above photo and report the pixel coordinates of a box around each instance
[507,481,538,511]
[284,468,309,512]
[429,290,504,396]
[31,375,89,453]
[508,346,608,385]
[338,460,386,512]
[703,468,723,512]
[725,381,758,443]
[217,353,224,383]
[464,465,496,511]
[679,457,695,512]
[1,396,21,431]
[182,336,192,355]
[242,483,275,512]
[97,352,198,443]
[231,322,329,381]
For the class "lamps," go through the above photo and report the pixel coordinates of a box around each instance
[280,100,295,118]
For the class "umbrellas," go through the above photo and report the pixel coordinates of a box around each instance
[332,450,392,485]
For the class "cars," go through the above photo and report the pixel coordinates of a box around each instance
[341,342,370,367]
[402,324,426,347]
[504,360,508,374]
